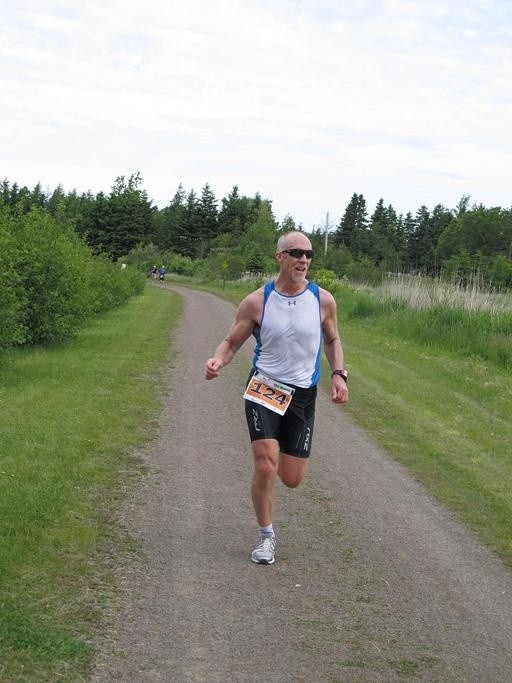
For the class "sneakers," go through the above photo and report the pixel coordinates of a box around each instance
[251,531,276,565]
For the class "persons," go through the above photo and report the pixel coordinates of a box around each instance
[151,265,159,280]
[203,230,351,567]
[159,264,166,288]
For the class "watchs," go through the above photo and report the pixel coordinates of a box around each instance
[331,368,348,382]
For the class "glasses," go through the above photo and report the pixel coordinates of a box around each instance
[281,248,315,259]
